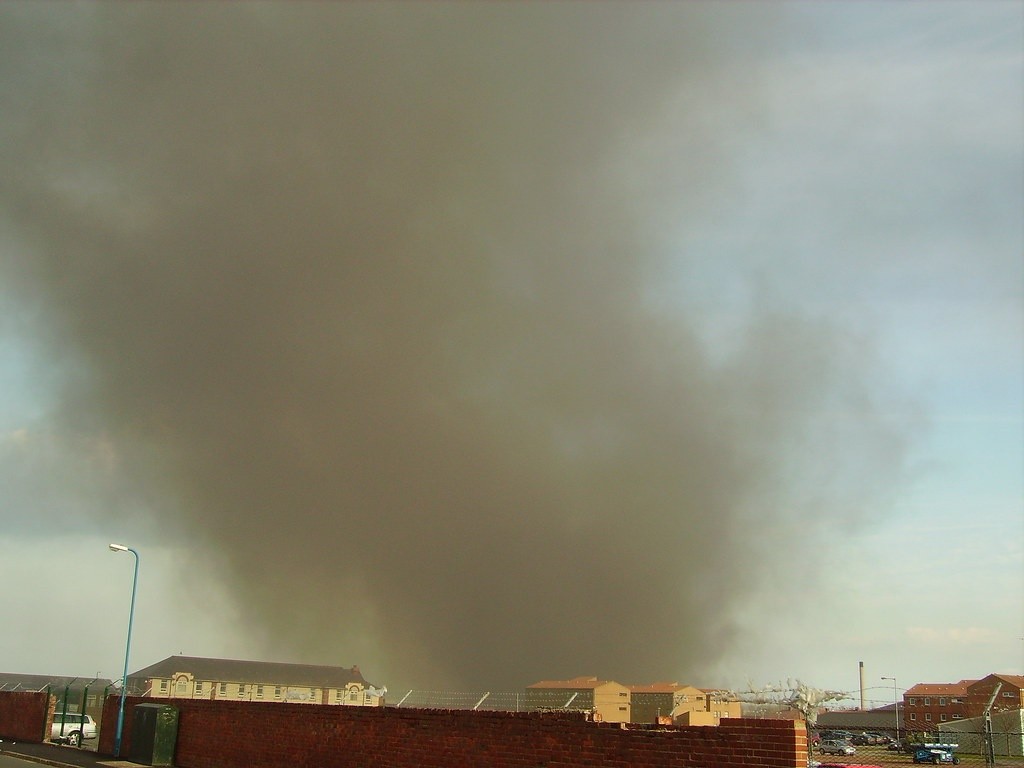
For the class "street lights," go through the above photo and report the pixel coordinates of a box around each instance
[109,544,139,759]
[881,677,901,754]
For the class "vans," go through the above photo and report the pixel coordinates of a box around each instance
[50,712,97,746]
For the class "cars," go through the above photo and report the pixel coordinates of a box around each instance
[817,740,856,757]
[811,726,905,751]
[914,749,960,764]
